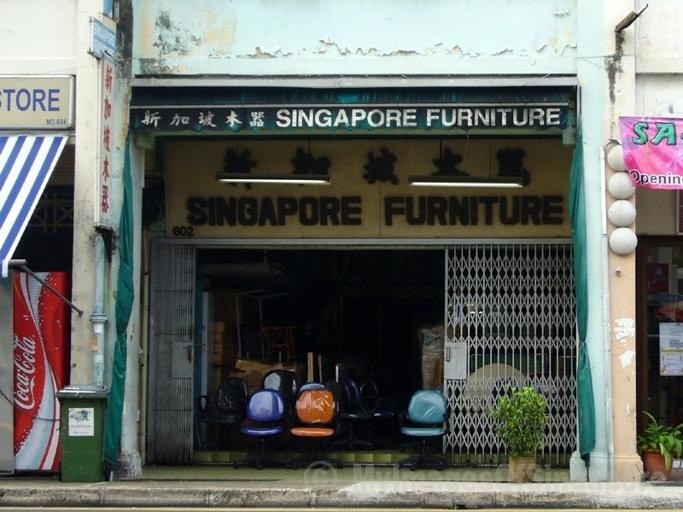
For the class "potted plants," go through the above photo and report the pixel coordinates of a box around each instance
[633,411,683,481]
[488,384,548,483]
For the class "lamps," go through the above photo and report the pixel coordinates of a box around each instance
[406,137,525,189]
[216,140,332,188]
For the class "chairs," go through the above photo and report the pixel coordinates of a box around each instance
[195,369,387,466]
[399,388,450,471]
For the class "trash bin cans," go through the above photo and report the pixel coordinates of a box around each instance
[56,383,109,481]
